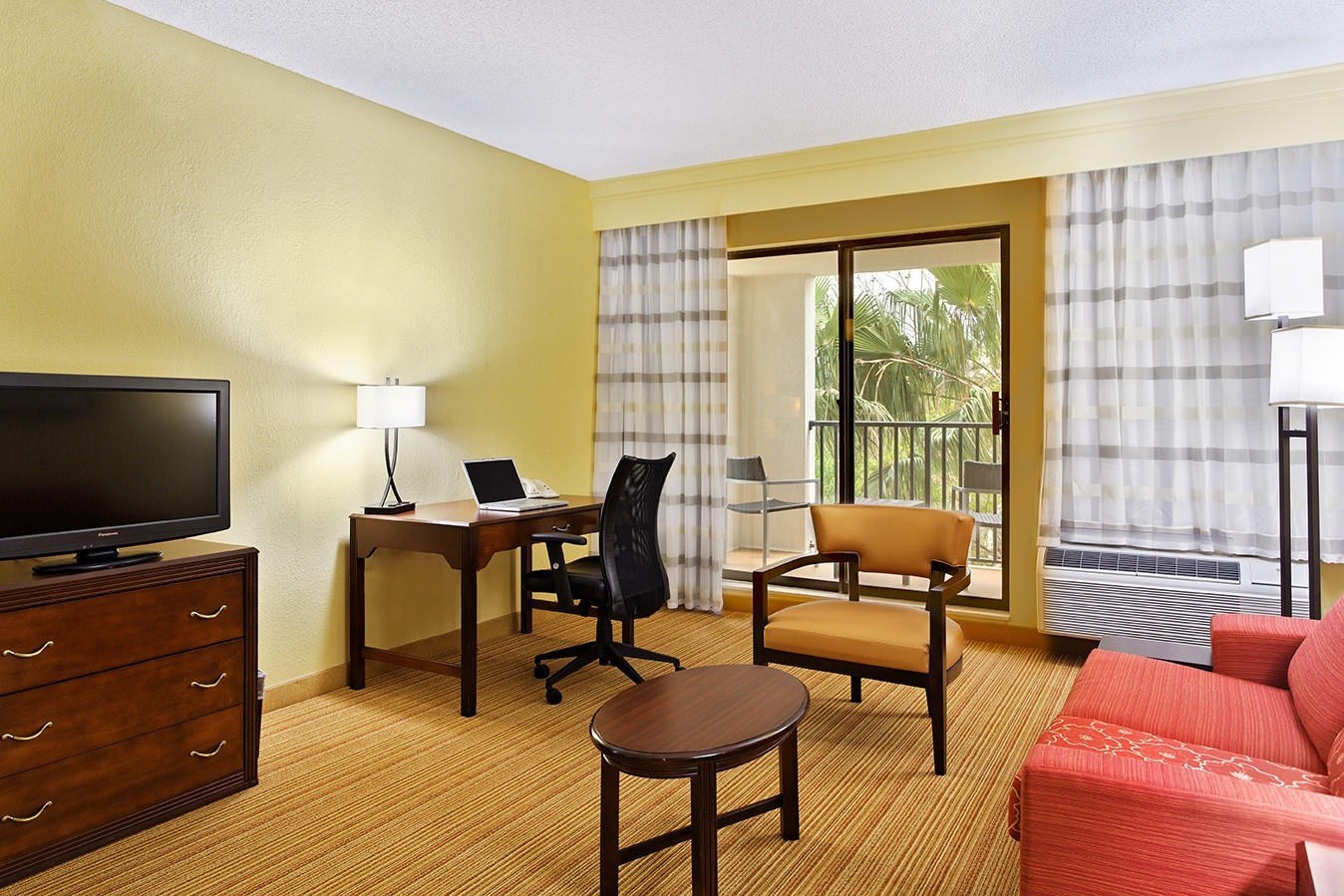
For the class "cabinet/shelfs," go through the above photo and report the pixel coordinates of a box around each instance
[0,537,260,885]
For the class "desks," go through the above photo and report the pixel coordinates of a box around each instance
[347,494,602,718]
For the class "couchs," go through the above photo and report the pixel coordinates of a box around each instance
[1008,597,1344,896]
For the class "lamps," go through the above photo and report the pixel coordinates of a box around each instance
[1244,235,1343,620]
[357,376,425,512]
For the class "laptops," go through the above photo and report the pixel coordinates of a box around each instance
[460,457,569,512]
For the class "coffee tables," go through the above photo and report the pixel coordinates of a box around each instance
[592,665,810,896]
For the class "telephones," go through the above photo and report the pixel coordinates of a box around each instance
[520,478,559,498]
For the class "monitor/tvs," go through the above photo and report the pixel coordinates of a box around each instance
[0,372,231,574]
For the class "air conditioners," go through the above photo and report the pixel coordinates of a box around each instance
[1036,540,1320,648]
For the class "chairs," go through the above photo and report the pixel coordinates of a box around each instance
[753,505,975,775]
[525,452,687,704]
[727,457,823,566]
[951,458,1002,559]
